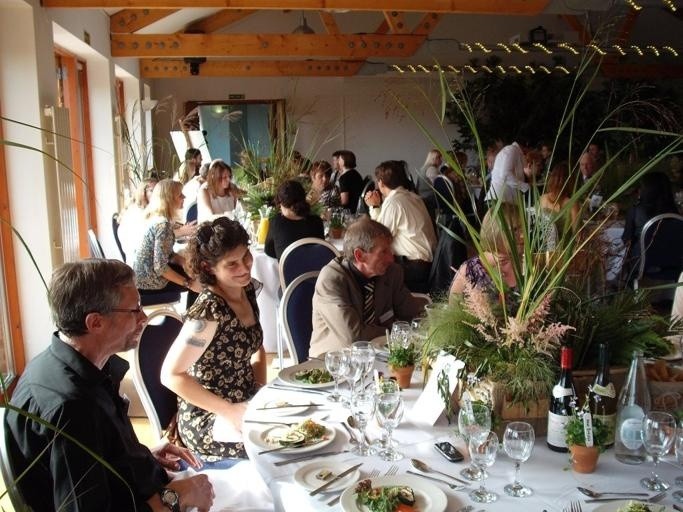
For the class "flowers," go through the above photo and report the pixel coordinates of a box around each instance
[368,40,682,413]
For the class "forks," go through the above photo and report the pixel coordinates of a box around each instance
[584,488,667,503]
[561,498,582,512]
[326,465,398,505]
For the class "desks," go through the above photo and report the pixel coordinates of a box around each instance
[241,345,678,511]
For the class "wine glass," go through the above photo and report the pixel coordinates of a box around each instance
[390,317,428,347]
[640,410,676,493]
[458,402,535,503]
[324,340,403,461]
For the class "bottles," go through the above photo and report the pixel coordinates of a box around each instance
[257,207,271,246]
[327,206,352,231]
[614,348,650,464]
[582,341,617,448]
[547,344,581,453]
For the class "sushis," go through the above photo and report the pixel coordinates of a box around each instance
[398,486,415,506]
[279,431,305,447]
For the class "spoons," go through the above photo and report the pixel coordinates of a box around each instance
[406,470,466,492]
[577,484,649,498]
[410,458,470,490]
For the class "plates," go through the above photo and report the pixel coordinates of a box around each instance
[371,336,394,354]
[339,474,447,512]
[247,416,336,453]
[292,460,360,492]
[249,357,346,415]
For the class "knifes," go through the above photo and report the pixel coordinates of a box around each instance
[273,450,351,466]
[307,462,364,496]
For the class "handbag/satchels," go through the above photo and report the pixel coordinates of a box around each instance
[161,416,188,472]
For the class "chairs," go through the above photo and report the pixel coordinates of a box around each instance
[0,409,43,512]
[87,163,682,446]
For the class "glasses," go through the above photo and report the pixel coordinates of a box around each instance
[198,215,231,252]
[81,305,143,319]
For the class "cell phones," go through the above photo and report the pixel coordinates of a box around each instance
[434,440,465,462]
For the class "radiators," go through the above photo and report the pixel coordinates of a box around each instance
[43,106,82,264]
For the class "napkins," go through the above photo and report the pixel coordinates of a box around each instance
[173,454,277,511]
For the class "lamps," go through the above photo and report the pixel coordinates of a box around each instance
[289,11,316,35]
